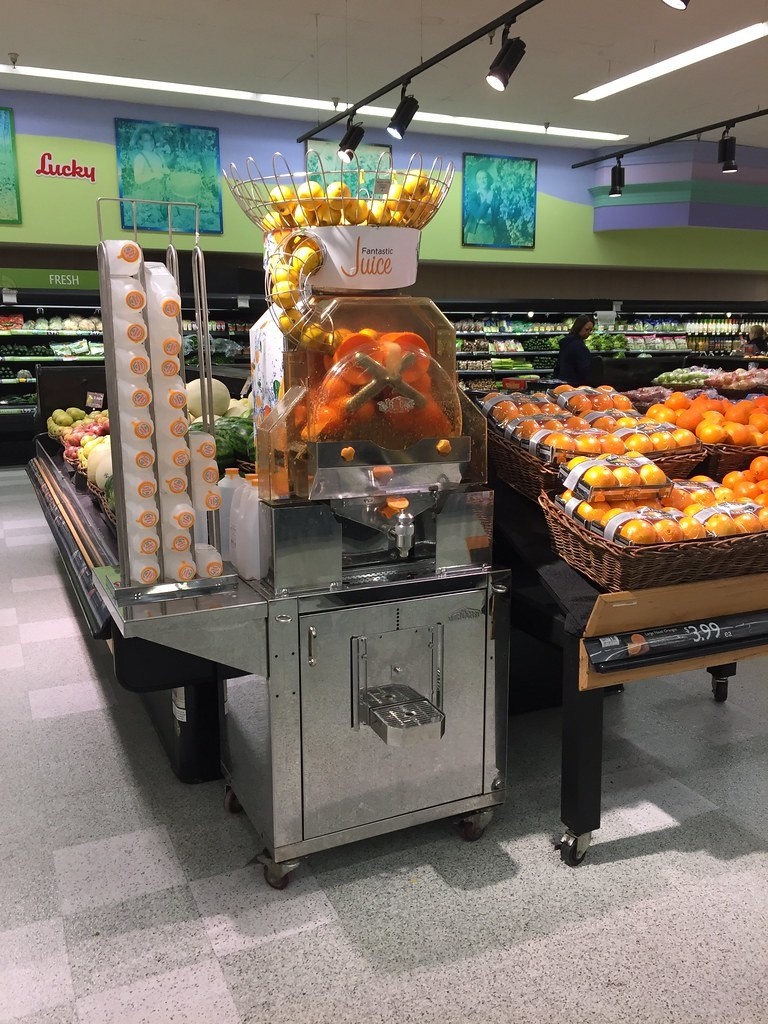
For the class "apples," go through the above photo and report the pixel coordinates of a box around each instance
[654,367,768,390]
[62,413,109,472]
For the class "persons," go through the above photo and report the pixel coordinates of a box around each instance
[463,168,512,245]
[740,325,768,351]
[557,315,603,388]
[134,128,170,221]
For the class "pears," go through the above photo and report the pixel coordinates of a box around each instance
[47,408,109,437]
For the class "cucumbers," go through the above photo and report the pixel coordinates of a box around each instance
[0,343,54,379]
[523,336,560,369]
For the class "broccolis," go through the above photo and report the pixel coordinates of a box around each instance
[24,315,102,331]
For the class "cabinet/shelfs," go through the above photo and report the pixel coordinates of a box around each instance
[0,298,768,470]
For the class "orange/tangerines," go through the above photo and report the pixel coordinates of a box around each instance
[258,170,443,439]
[481,383,768,546]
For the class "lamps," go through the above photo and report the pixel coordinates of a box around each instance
[338,111,366,163]
[486,22,525,91]
[609,156,624,198]
[386,75,418,139]
[719,121,738,175]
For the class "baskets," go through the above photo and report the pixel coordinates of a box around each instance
[483,405,768,594]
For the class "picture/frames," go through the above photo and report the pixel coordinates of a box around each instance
[459,153,537,248]
[113,117,222,238]
[0,106,22,224]
[305,139,393,195]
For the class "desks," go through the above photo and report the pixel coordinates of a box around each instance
[492,496,768,856]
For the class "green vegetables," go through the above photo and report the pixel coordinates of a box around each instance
[490,356,540,387]
[550,332,629,359]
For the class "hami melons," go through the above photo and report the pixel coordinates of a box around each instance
[185,377,255,424]
[88,440,114,490]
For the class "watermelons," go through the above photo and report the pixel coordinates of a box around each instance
[105,409,255,518]
